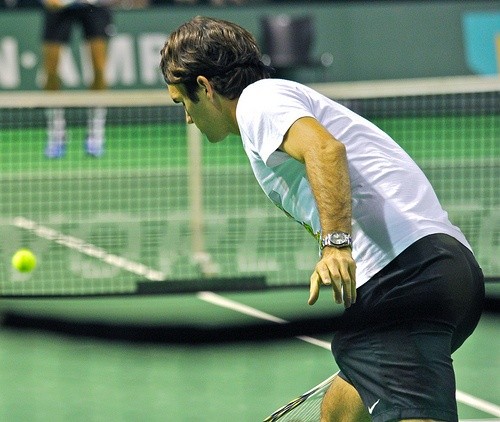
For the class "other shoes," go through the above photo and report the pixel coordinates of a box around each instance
[86,132,103,157]
[43,135,66,157]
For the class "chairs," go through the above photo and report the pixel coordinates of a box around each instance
[256,12,334,82]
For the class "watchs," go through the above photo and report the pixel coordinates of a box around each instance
[321,232,354,251]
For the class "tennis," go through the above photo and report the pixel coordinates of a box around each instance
[13,250,36,272]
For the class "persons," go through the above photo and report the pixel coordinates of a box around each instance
[159,16,485,422]
[38,0,148,157]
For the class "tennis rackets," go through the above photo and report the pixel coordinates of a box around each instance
[263,370,340,422]
